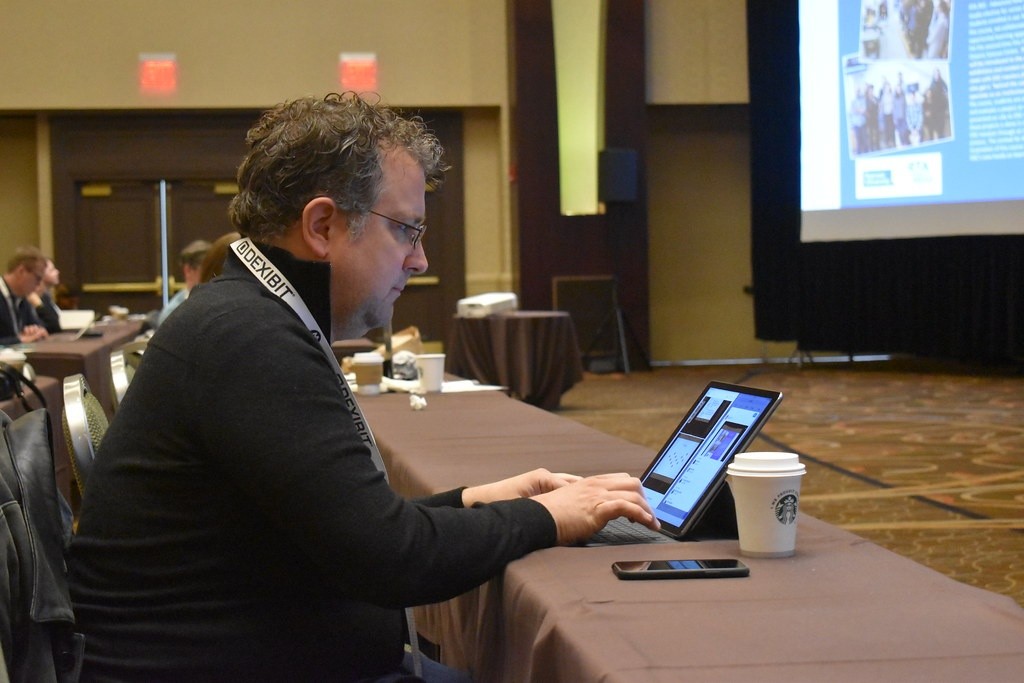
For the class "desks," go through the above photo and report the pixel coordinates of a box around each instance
[349,384,1024,683]
[8,318,146,424]
[0,374,72,512]
[450,313,585,413]
[330,338,376,371]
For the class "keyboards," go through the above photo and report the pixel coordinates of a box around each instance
[582,515,679,547]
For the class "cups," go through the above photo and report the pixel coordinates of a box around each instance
[0,350,27,377]
[352,352,385,395]
[726,452,807,558]
[114,307,128,326]
[414,353,446,394]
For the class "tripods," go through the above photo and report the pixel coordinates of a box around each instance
[581,203,652,378]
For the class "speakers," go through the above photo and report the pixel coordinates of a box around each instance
[597,149,643,202]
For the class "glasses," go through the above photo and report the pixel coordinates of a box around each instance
[303,194,427,250]
[33,273,42,282]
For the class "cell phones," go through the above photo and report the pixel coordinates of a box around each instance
[611,559,750,581]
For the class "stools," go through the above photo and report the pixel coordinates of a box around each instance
[790,346,815,370]
[734,284,781,386]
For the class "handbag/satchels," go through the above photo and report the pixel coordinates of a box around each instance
[0,361,20,401]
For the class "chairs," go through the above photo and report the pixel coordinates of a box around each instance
[61,375,109,501]
[109,349,141,411]
[111,341,150,407]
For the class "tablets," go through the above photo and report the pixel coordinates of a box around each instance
[641,380,784,537]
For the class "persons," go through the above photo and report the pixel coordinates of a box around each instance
[67,91,662,682]
[849,1,951,155]
[0,230,242,348]
